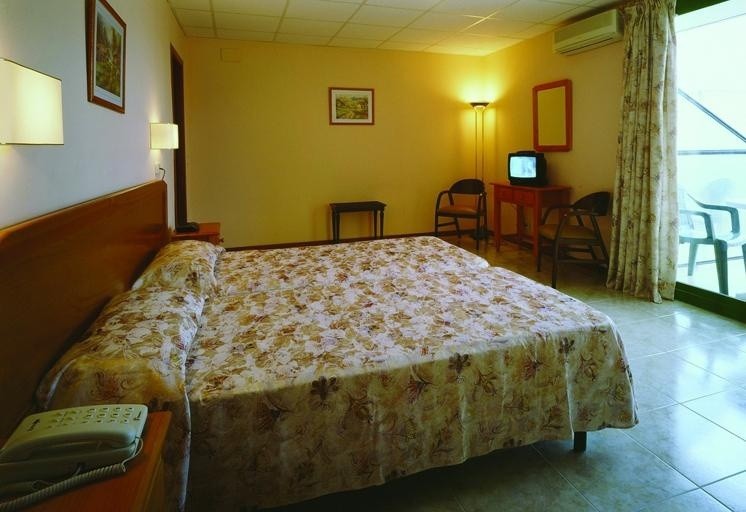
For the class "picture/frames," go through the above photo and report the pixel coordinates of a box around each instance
[85,0,127,114]
[328,86,375,126]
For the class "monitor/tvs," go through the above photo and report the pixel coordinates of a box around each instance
[506,152,546,184]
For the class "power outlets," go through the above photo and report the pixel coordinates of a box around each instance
[155,163,160,175]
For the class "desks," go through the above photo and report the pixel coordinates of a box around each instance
[329,201,387,243]
[489,181,571,273]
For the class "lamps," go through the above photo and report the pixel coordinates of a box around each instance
[0,58,65,147]
[470,99,489,211]
[149,122,179,150]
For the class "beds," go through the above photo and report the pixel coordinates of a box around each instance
[0,174,638,512]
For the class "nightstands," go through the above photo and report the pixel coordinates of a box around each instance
[0,411,173,512]
[172,222,224,247]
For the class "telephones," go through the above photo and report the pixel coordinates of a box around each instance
[0,403,148,504]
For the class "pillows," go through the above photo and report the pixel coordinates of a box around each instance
[130,238,225,294]
[33,286,205,409]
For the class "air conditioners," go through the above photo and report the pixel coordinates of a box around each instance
[551,8,624,56]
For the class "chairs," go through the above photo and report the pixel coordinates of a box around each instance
[434,179,487,251]
[676,186,746,297]
[539,189,612,291]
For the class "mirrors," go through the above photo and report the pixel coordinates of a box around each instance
[532,79,573,153]
